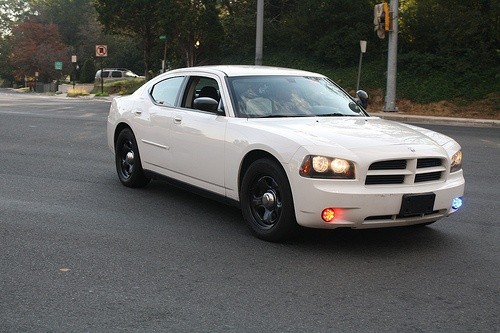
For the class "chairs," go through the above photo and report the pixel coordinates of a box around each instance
[199,86,220,101]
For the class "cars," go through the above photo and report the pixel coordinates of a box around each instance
[106,64,466,242]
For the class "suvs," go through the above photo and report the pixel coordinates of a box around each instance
[95,68,145,84]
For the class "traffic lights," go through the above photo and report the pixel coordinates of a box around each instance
[378,3,390,32]
